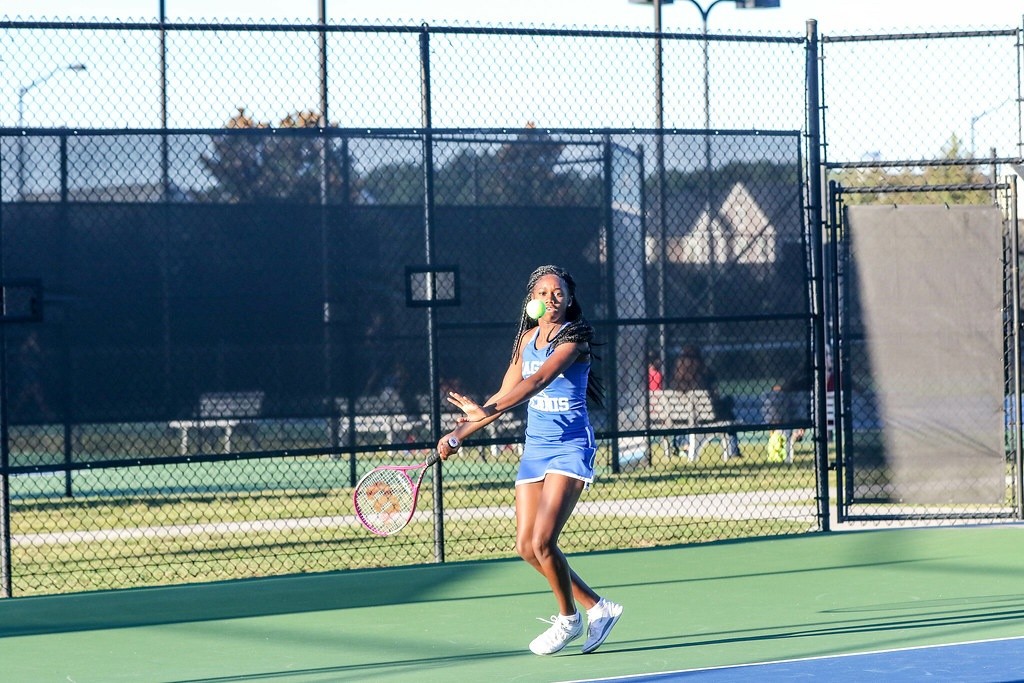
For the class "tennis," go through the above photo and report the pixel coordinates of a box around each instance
[525,299,546,319]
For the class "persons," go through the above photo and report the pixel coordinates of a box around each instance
[437,266,623,656]
[671,344,744,457]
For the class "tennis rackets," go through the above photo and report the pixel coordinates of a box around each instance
[353,436,459,536]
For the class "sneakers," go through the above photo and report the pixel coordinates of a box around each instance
[529,614,584,655]
[582,599,623,654]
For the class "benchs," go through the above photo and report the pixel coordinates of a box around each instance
[168,391,264,454]
[647,389,844,466]
[328,397,523,458]
